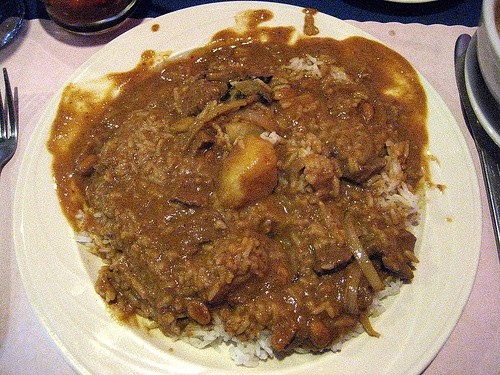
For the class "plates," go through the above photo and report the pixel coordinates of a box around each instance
[13,0,481,375]
[464,30,500,147]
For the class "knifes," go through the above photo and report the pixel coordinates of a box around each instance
[455,35,500,248]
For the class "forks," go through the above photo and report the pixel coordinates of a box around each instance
[0,68,19,169]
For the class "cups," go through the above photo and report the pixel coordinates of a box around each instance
[0,0,24,49]
[43,0,136,35]
[478,0,500,103]
